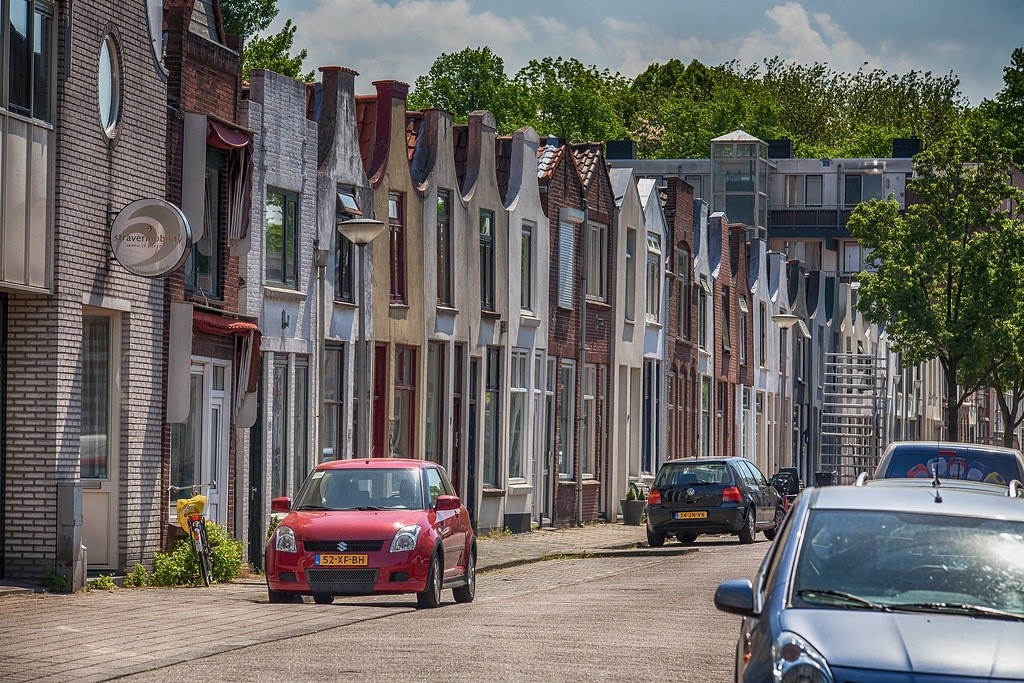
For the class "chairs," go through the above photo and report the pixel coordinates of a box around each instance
[359,491,369,499]
[337,481,360,501]
[721,473,730,483]
[400,479,415,504]
[677,474,697,483]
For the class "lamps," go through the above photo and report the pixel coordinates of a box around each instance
[914,379,923,389]
[893,374,902,385]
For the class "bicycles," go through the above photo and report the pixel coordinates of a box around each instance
[165,480,218,588]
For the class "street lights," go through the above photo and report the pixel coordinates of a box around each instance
[772,313,799,466]
[336,216,388,459]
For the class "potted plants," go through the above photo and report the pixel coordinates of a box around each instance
[619,484,648,527]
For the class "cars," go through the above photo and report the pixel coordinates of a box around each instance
[812,471,1024,581]
[870,441,1024,490]
[263,457,481,610]
[645,455,790,546]
[714,481,1023,683]
